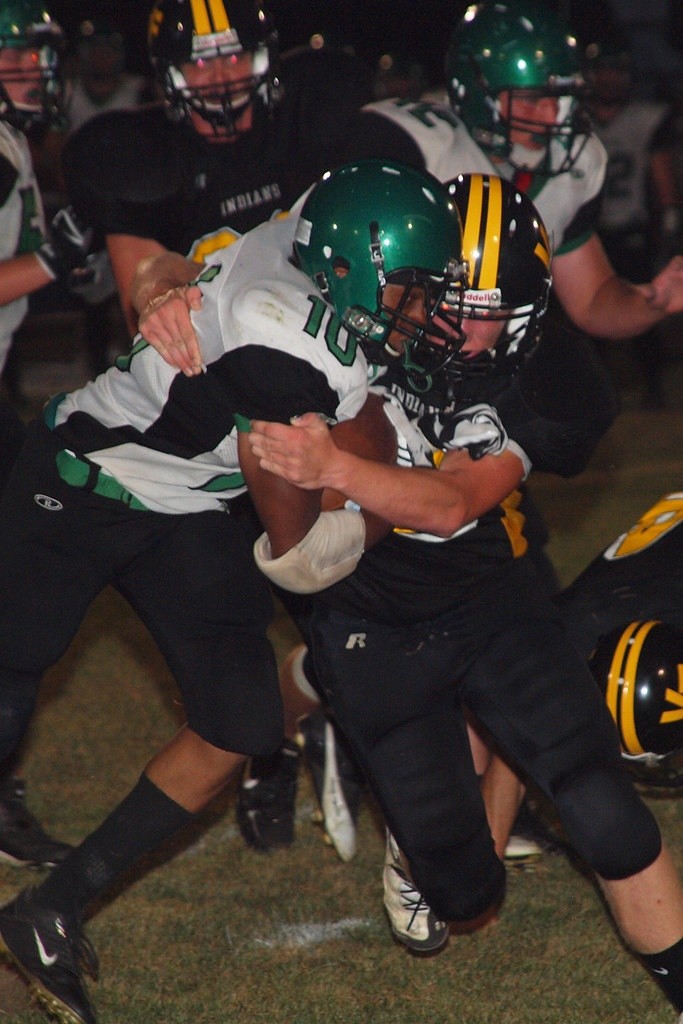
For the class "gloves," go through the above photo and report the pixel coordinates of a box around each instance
[36,203,99,277]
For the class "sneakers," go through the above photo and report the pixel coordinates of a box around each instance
[500,823,549,866]
[0,887,98,1023]
[0,774,74,870]
[236,738,301,853]
[305,714,365,862]
[383,824,451,958]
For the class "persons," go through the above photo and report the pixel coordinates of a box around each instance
[0,1,683,1024]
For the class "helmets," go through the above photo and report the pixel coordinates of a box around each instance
[446,0,596,179]
[433,172,559,387]
[594,617,683,798]
[0,0,72,133]
[149,0,280,155]
[284,156,467,368]
